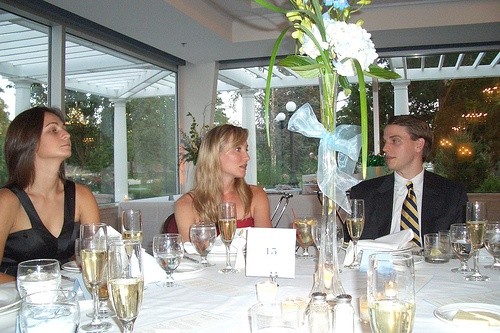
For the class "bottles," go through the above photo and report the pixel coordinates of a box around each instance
[309,291,355,333]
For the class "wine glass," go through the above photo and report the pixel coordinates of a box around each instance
[75,209,148,333]
[448,201,500,282]
[152,199,368,290]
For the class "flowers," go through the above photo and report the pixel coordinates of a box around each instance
[254,0,401,214]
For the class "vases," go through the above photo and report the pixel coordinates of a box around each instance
[303,73,356,322]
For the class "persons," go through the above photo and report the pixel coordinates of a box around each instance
[0,108,99,285]
[345,114,468,248]
[174,125,273,246]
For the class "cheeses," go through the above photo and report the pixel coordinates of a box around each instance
[451,310,489,327]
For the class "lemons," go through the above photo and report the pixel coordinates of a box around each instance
[430,248,442,256]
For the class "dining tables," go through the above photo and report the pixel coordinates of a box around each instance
[0,241,500,333]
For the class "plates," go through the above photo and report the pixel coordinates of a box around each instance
[341,240,420,261]
[61,261,81,273]
[0,284,22,316]
[434,303,500,328]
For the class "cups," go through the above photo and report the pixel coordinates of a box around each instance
[19,290,80,333]
[423,230,459,263]
[16,259,61,304]
[367,253,416,333]
[359,296,370,326]
[251,278,308,333]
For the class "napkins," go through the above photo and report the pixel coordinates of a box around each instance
[354,228,416,252]
[184,226,252,258]
[86,227,174,285]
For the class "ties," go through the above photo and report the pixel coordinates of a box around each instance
[400,181,421,249]
